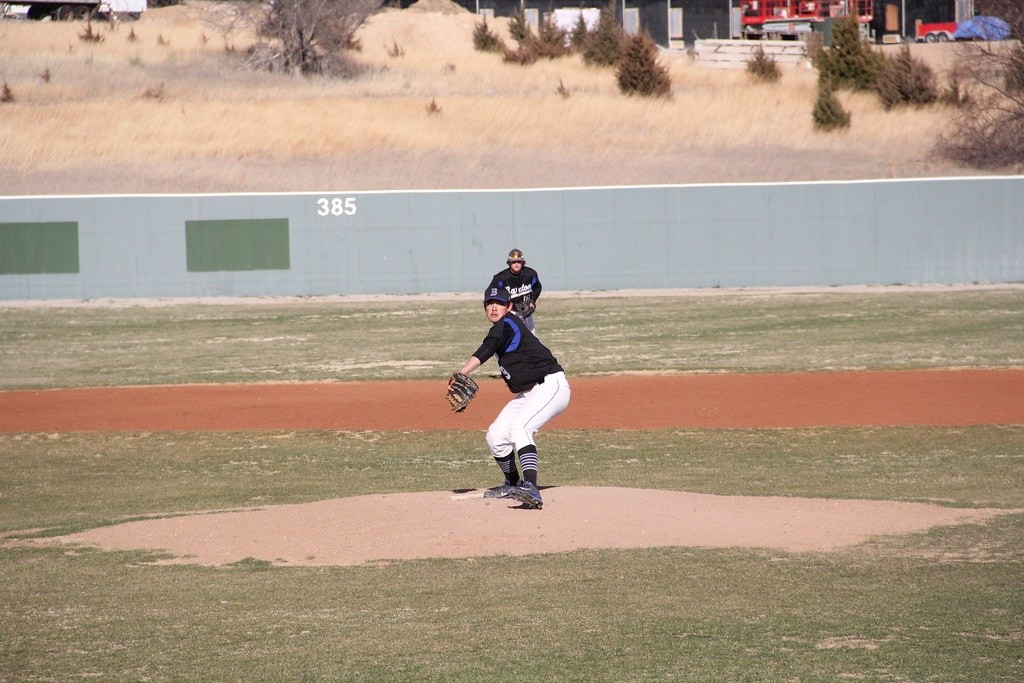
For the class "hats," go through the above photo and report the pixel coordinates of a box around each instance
[483,286,509,301]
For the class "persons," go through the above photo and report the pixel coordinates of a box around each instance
[488,250,542,338]
[448,283,573,510]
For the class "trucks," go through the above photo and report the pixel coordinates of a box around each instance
[0,0,147,21]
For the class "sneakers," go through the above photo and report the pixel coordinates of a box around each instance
[483,479,523,499]
[507,480,543,509]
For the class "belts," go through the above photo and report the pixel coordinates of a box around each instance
[538,377,544,385]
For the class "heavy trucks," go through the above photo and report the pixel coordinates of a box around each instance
[740,0,875,41]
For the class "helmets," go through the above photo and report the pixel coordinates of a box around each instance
[507,249,525,265]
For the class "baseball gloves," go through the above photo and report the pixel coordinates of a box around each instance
[445,372,479,413]
[519,307,535,318]
